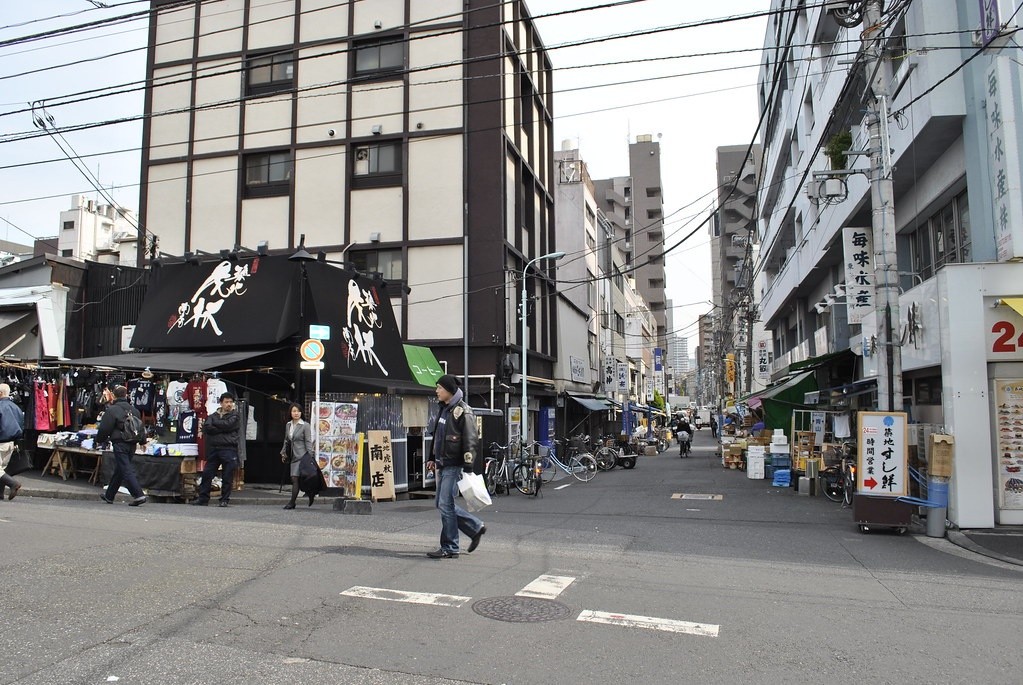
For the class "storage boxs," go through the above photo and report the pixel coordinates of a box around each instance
[645,445,656,455]
[168,443,200,455]
[52,451,70,476]
[180,461,197,474]
[926,433,953,477]
[720,416,822,488]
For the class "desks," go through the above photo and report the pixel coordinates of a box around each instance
[794,470,832,496]
[101,451,199,505]
[37,444,102,486]
[852,492,915,535]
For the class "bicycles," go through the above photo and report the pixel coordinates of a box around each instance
[484,441,518,499]
[536,438,598,484]
[576,434,619,471]
[512,440,544,500]
[654,434,670,453]
[679,430,694,458]
[820,442,857,507]
[696,423,701,431]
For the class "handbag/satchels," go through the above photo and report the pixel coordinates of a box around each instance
[457,468,492,512]
[6,444,33,476]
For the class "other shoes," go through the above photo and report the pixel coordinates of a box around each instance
[220,502,227,507]
[191,500,208,506]
[308,494,315,506]
[100,494,113,504]
[129,496,146,506]
[284,503,295,509]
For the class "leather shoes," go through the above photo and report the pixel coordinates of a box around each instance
[468,527,486,552]
[426,549,459,558]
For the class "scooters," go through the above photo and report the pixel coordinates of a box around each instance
[594,437,639,469]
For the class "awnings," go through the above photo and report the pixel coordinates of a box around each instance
[803,377,878,404]
[564,390,613,411]
[771,347,851,382]
[734,371,818,410]
[38,346,292,406]
[608,398,666,416]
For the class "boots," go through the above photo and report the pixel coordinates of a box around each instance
[0,473,21,500]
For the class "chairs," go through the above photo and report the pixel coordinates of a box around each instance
[796,431,816,470]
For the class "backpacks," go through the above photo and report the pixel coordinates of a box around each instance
[114,404,144,442]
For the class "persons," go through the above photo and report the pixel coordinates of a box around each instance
[193,392,241,507]
[95,385,147,506]
[676,418,693,455]
[0,383,24,500]
[426,374,487,558]
[280,402,315,509]
[710,418,718,437]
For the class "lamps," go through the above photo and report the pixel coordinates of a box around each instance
[317,248,326,261]
[375,20,380,27]
[189,250,221,266]
[814,273,876,314]
[258,239,272,256]
[289,234,317,262]
[370,232,380,241]
[372,124,383,135]
[152,250,176,268]
[347,262,388,287]
[226,244,257,262]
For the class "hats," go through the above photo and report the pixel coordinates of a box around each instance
[436,375,457,395]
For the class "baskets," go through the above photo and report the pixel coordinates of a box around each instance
[822,448,841,466]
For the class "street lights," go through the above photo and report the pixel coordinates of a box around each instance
[522,252,567,489]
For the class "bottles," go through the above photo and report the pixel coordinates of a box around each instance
[774,466,790,487]
